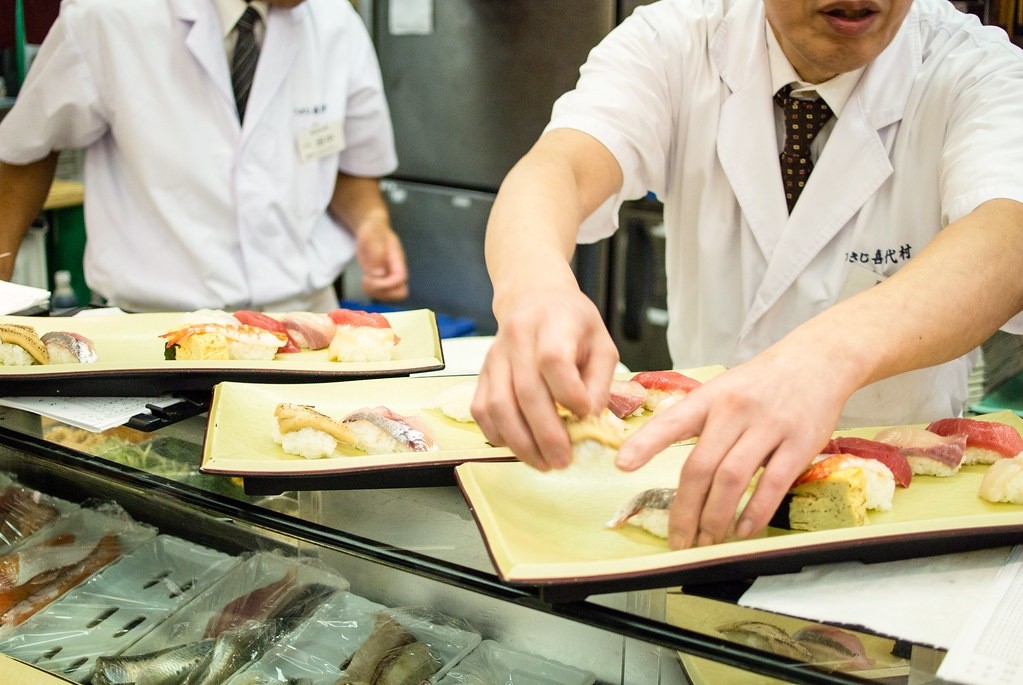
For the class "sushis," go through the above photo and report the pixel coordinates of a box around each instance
[0,324,99,368]
[273,370,706,460]
[609,409,1023,543]
[162,307,403,363]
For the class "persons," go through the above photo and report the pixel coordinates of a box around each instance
[0,0,414,305]
[464,0,1023,549]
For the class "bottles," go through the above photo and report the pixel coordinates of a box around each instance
[52,271,77,311]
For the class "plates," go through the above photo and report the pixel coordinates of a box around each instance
[455,409,1023,585]
[0,280,52,315]
[0,309,446,378]
[0,475,597,685]
[199,364,730,477]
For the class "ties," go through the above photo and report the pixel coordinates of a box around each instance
[230,5,261,126]
[774,83,834,216]
